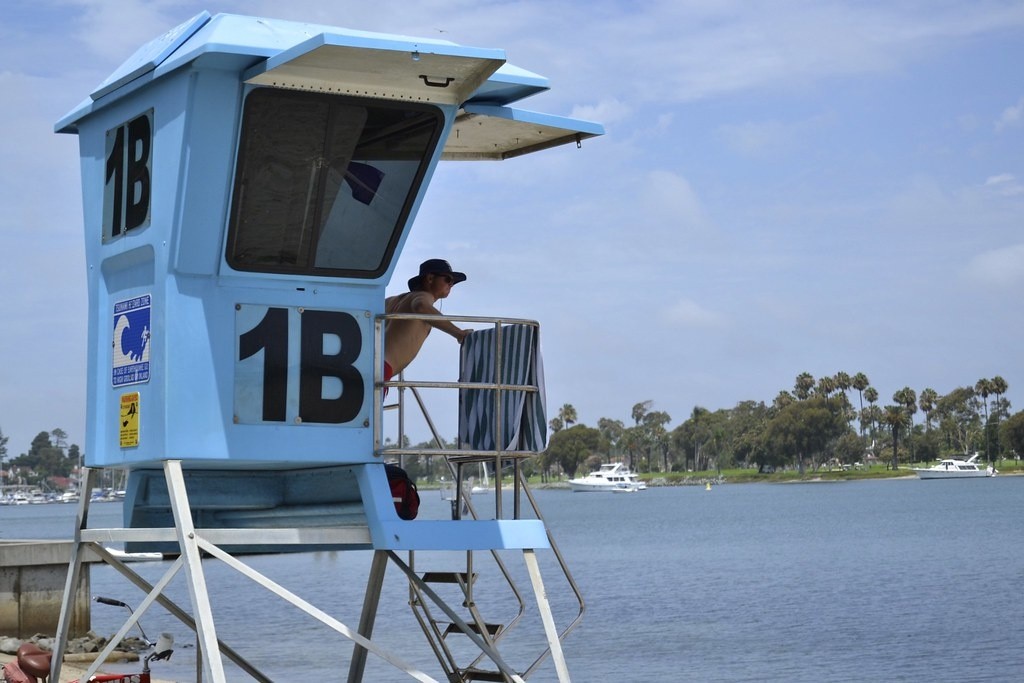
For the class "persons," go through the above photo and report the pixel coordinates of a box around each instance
[381,258,474,398]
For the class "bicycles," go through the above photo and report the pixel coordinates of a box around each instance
[0,595,175,683]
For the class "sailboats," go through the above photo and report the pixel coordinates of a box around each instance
[0,452,128,507]
[471,461,490,494]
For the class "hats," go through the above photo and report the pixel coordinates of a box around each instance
[408,259,467,292]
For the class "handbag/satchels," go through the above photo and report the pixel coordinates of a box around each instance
[386,464,420,520]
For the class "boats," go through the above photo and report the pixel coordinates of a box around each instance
[563,462,648,492]
[912,451,987,479]
[611,483,637,493]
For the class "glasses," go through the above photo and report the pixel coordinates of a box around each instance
[435,275,454,288]
